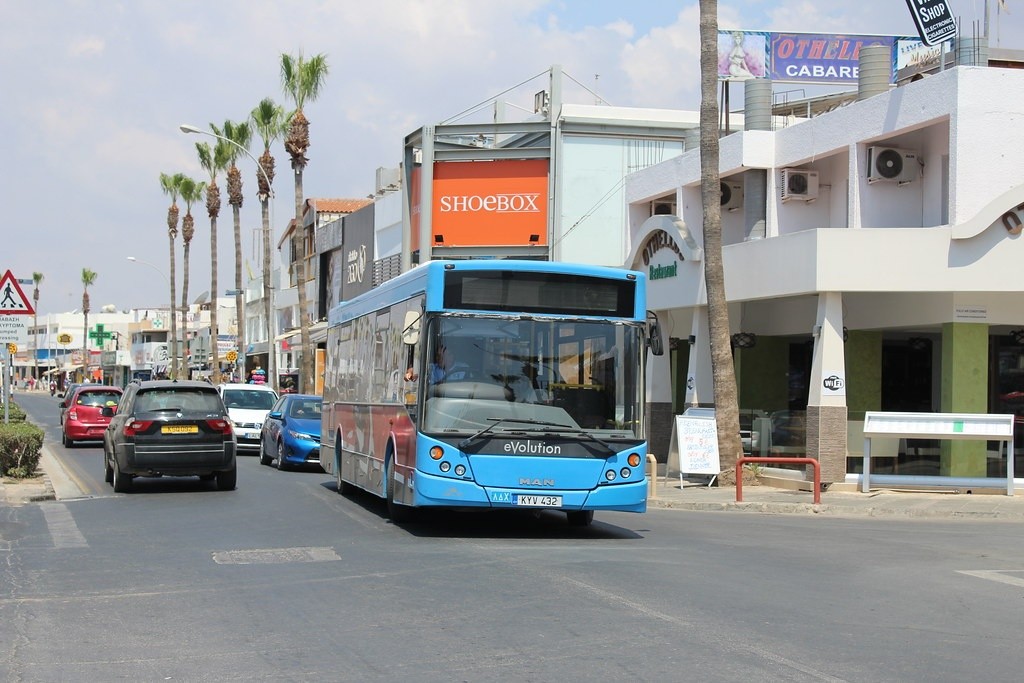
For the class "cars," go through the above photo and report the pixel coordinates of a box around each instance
[57,384,107,425]
[216,382,280,452]
[259,394,323,470]
[58,386,124,448]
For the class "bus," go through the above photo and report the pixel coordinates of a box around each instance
[319,260,647,528]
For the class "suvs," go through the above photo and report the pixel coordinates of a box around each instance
[101,379,237,493]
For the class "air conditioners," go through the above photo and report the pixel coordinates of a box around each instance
[780,168,820,200]
[866,146,920,182]
[720,180,743,210]
[652,200,677,215]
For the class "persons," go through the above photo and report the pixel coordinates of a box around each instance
[22,375,102,394]
[403,342,475,384]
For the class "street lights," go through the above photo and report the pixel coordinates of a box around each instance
[127,256,174,380]
[180,123,281,393]
[32,271,44,389]
[82,269,95,382]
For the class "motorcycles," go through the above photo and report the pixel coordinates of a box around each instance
[50,383,57,397]
